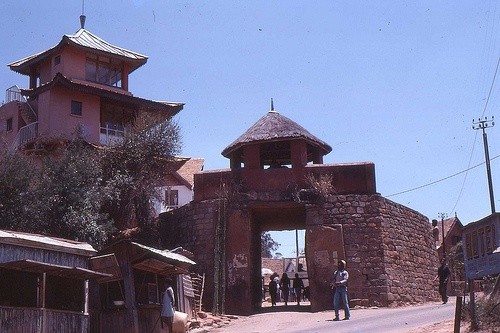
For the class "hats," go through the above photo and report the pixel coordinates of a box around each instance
[341,259,346,269]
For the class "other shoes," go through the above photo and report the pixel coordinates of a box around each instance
[333,317,339,320]
[343,317,349,320]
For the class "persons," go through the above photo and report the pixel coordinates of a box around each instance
[433,258,450,304]
[331,260,350,320]
[293,273,305,306]
[161,278,175,333]
[280,273,290,307]
[432,219,439,241]
[269,275,277,306]
